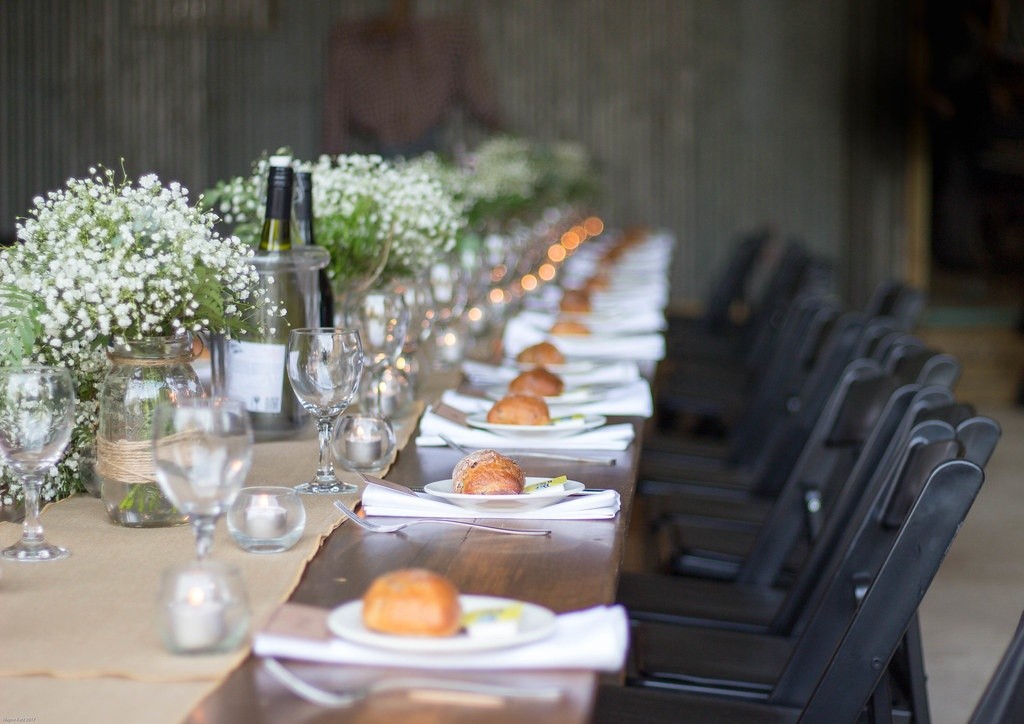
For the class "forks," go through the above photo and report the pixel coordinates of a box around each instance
[436,434,616,466]
[333,498,552,535]
[266,654,562,705]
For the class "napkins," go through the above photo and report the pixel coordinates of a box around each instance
[363,473,621,519]
[254,603,628,672]
[415,408,635,452]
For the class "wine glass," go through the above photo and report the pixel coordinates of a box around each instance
[0,200,624,664]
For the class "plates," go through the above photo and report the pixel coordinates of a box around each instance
[326,231,666,654]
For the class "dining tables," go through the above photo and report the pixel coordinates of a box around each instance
[188,417,640,724]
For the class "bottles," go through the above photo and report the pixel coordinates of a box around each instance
[241,164,309,433]
[286,171,335,362]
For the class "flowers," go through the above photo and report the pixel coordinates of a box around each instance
[0,143,586,506]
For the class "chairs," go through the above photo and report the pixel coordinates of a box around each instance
[594,227,1024,723]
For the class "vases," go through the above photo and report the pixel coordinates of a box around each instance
[80,432,105,494]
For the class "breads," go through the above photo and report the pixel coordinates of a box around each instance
[452,448,526,497]
[363,564,460,635]
[488,226,646,426]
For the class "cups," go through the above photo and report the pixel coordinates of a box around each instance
[219,244,332,446]
